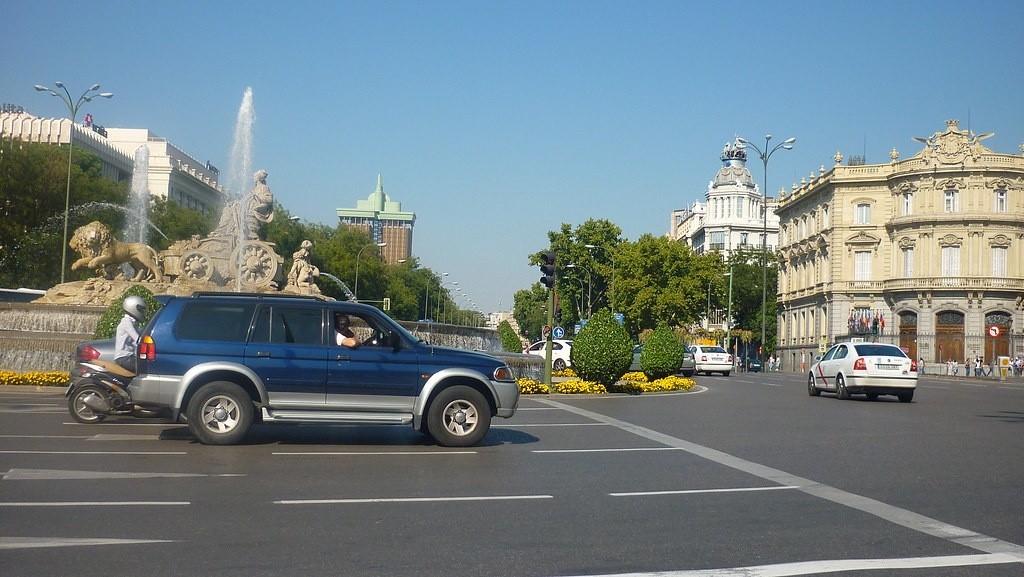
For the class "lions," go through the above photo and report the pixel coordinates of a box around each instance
[69,221,163,285]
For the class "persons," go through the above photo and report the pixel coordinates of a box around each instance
[953,360,958,375]
[768,356,780,371]
[244,169,273,214]
[974,359,994,377]
[334,314,360,347]
[292,239,315,283]
[965,358,970,377]
[919,358,924,375]
[115,296,147,375]
[1008,355,1024,374]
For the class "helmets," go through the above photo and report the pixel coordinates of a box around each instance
[122,296,147,322]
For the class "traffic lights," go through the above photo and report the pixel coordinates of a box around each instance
[556,308,562,322]
[540,251,556,288]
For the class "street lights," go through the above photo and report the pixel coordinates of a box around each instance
[354,243,387,300]
[707,273,730,339]
[567,265,591,320]
[562,277,583,320]
[35,80,114,284]
[585,244,615,340]
[735,134,795,372]
[424,272,483,327]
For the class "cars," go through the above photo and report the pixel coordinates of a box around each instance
[67,308,242,418]
[808,342,919,402]
[740,359,761,373]
[629,340,733,378]
[522,340,575,372]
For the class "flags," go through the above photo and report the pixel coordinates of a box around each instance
[848,309,885,329]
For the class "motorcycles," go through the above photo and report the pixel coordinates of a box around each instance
[65,359,137,424]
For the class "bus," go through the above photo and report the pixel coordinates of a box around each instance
[0,287,47,302]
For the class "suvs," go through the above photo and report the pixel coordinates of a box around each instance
[127,291,520,448]
